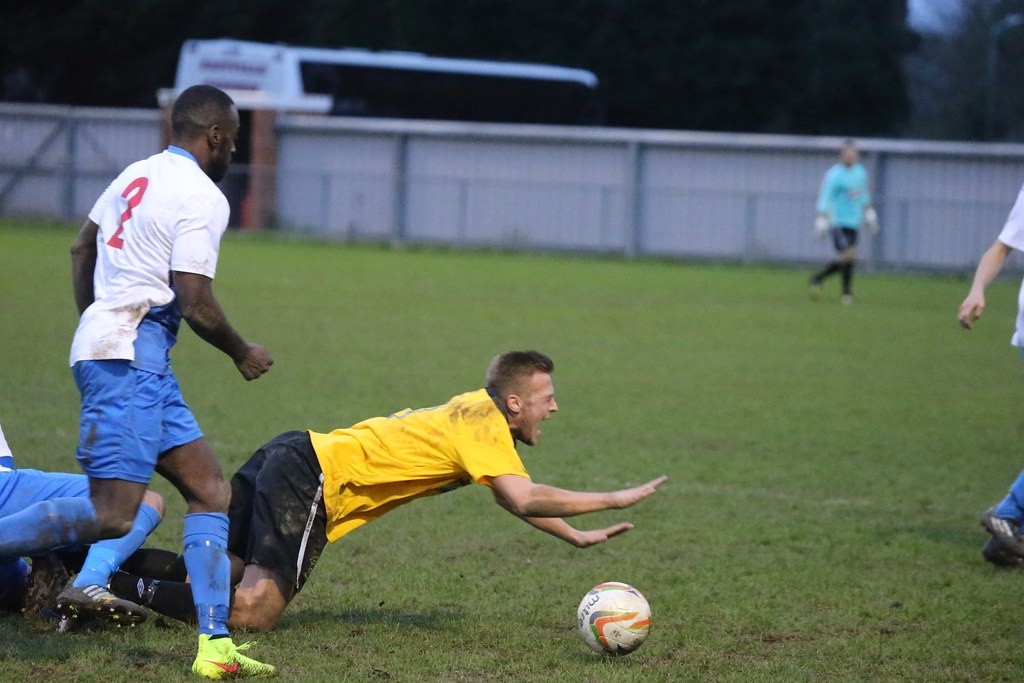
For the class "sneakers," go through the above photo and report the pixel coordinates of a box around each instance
[982,508,1024,557]
[24,553,60,619]
[56,583,148,626]
[57,607,94,633]
[190,633,277,681]
[982,533,1024,565]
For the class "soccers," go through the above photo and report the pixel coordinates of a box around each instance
[577,581,653,657]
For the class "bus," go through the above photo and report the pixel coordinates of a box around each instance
[155,28,606,167]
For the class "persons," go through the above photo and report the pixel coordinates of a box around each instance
[806,143,880,301]
[0,421,165,626]
[0,81,280,682]
[29,349,668,632]
[952,183,1024,567]
